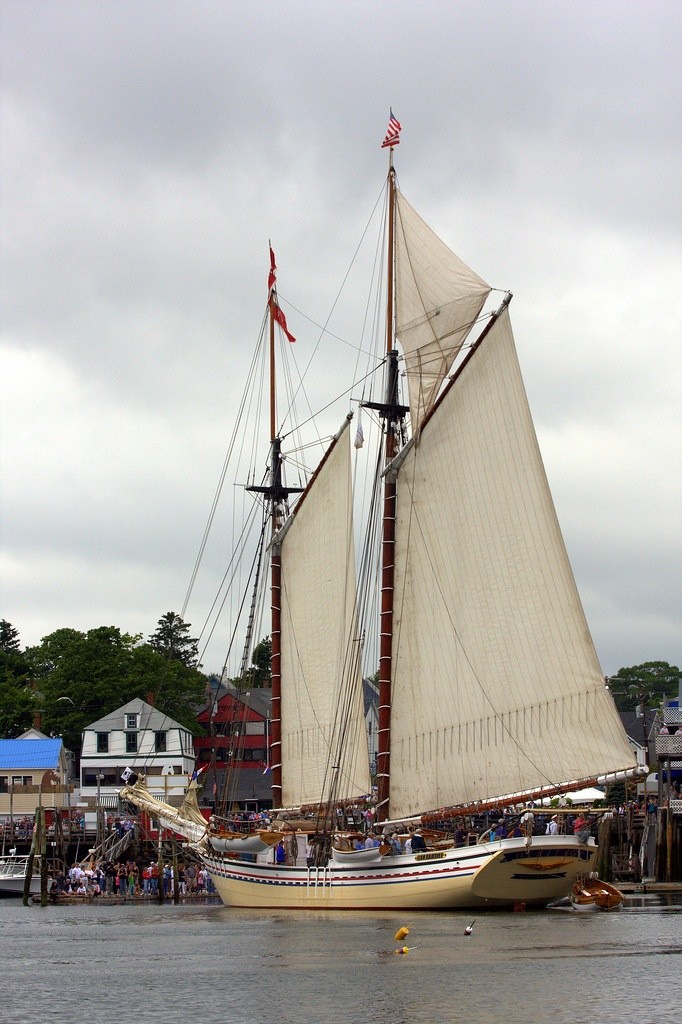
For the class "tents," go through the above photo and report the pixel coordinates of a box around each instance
[522,787,606,805]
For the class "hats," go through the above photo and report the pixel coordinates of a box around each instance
[551,815,557,819]
[416,829,422,832]
[368,832,375,835]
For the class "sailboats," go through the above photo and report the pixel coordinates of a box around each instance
[122,104,652,910]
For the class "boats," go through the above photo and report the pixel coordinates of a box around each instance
[0,853,56,896]
[567,876,624,912]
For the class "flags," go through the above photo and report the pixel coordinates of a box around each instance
[121,767,133,780]
[381,106,401,149]
[190,764,208,781]
[268,248,296,343]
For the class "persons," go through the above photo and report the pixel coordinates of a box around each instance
[0,722,682,898]
